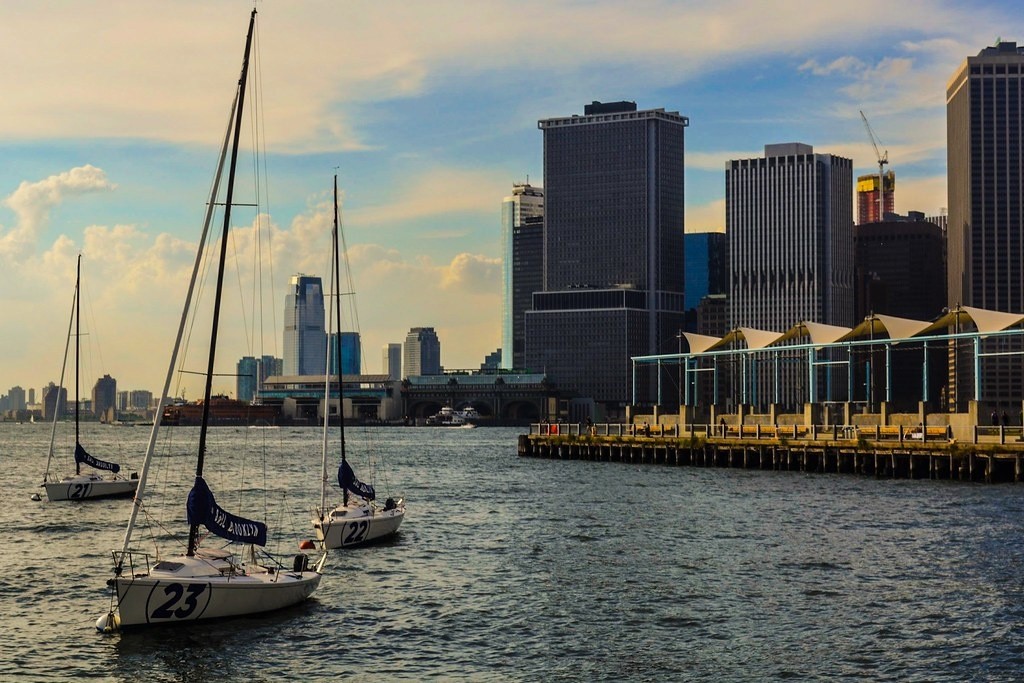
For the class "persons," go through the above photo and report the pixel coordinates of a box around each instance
[586,415,594,435]
[911,422,923,441]
[718,417,728,439]
[644,421,651,438]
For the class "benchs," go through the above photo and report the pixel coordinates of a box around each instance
[625,424,673,435]
[859,428,947,440]
[727,427,807,438]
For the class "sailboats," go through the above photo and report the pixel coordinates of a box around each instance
[108,20,330,634]
[100,410,107,424]
[108,407,113,424]
[308,173,406,552]
[29,415,34,424]
[38,253,140,502]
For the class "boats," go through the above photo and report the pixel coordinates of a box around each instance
[161,389,280,425]
[115,421,135,427]
[136,422,153,426]
[426,408,486,427]
[442,416,467,427]
[16,421,22,424]
